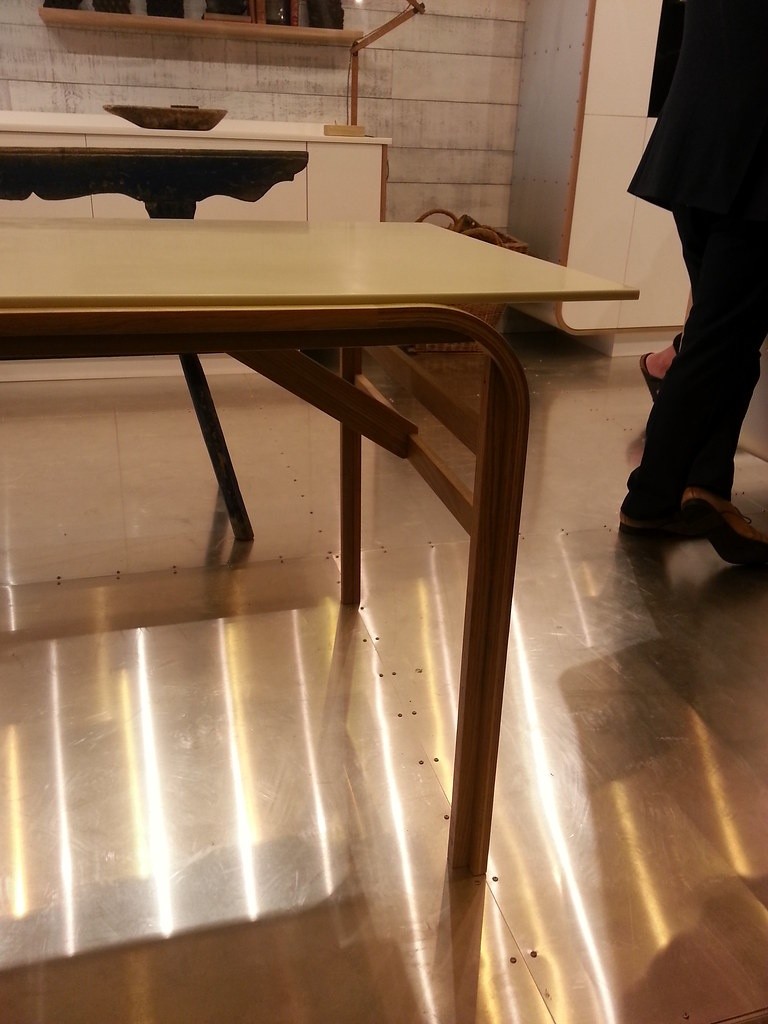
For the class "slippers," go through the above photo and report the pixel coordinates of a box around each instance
[640,351,664,404]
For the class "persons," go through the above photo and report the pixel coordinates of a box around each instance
[638,330,686,404]
[620,0,768,565]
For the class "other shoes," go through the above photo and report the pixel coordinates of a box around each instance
[618,485,767,565]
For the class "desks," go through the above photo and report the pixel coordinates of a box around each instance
[2,215,640,881]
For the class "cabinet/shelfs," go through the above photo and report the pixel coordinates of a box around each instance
[0,117,393,383]
